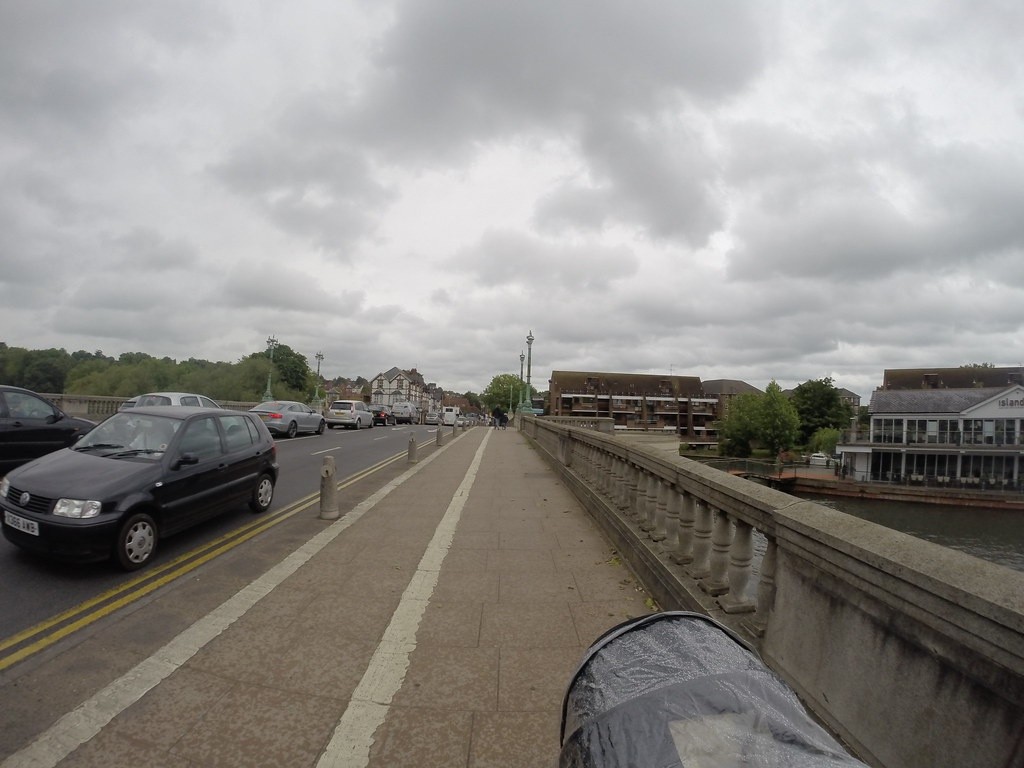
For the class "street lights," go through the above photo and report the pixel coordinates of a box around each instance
[517,350,526,408]
[522,329,534,409]
[313,350,324,400]
[261,334,279,403]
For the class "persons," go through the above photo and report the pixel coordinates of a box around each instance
[129,419,166,458]
[492,404,508,430]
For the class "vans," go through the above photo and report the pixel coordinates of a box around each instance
[392,402,421,425]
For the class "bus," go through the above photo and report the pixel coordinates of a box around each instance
[442,407,457,425]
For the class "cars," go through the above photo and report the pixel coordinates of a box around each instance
[457,417,470,426]
[0,385,109,467]
[133,393,242,437]
[1,407,280,570]
[368,405,397,426]
[325,400,375,430]
[116,393,142,413]
[249,401,326,439]
[425,413,441,425]
